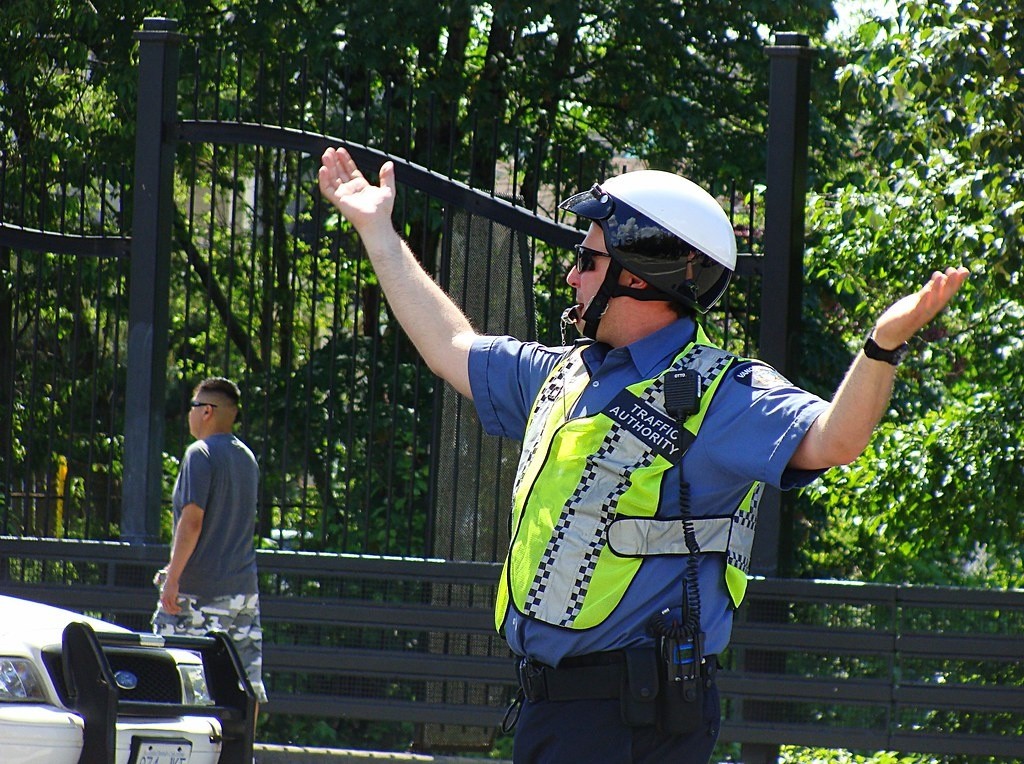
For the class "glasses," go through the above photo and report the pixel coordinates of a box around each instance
[574,244,611,274]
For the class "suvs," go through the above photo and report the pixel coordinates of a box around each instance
[0,594,259,764]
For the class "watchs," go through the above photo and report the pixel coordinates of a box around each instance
[862,325,910,366]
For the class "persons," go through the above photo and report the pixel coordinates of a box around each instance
[153,377,268,764]
[314,136,970,760]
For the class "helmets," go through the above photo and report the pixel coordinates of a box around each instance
[557,169,737,314]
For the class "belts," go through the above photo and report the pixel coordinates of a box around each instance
[518,660,668,705]
